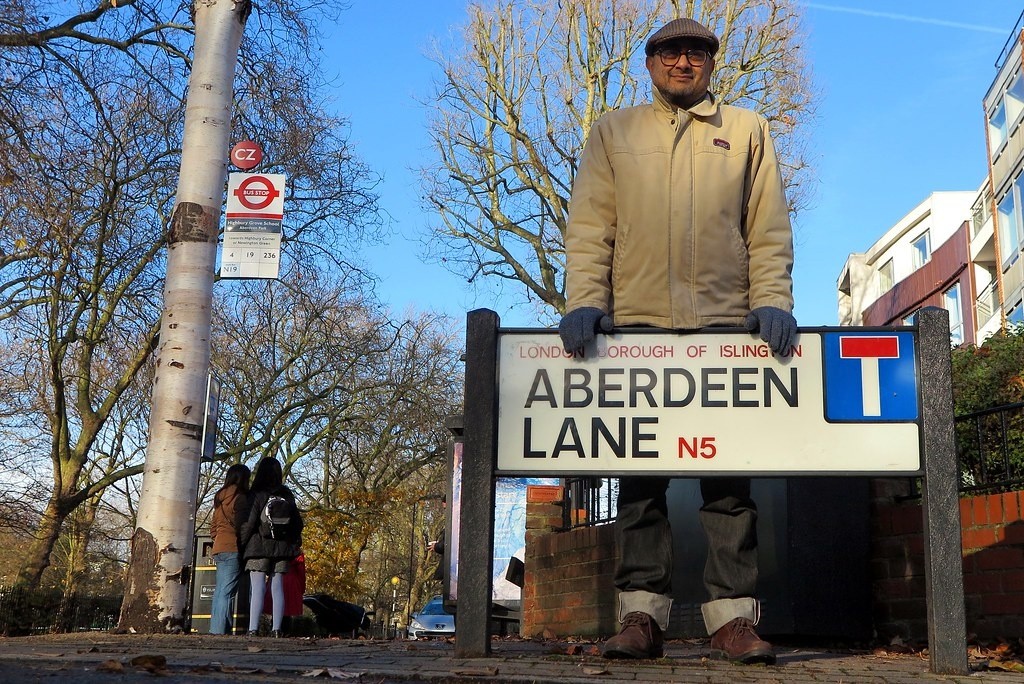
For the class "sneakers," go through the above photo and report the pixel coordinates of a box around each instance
[710,618,776,663]
[602,612,663,658]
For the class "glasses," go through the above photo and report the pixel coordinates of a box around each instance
[653,47,712,67]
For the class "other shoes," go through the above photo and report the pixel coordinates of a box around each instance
[271,630,281,638]
[246,630,258,637]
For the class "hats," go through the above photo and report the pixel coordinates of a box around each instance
[645,18,719,58]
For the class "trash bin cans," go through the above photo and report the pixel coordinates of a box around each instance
[188,533,249,635]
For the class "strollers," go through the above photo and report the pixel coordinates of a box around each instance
[300,592,376,640]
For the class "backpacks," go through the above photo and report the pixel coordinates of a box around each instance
[260,493,290,540]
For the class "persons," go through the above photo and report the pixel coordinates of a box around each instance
[236,456,303,639]
[209,464,251,636]
[557,18,798,663]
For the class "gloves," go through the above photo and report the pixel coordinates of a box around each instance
[747,306,797,356]
[559,307,613,353]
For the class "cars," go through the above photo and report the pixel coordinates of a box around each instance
[407,596,455,641]
